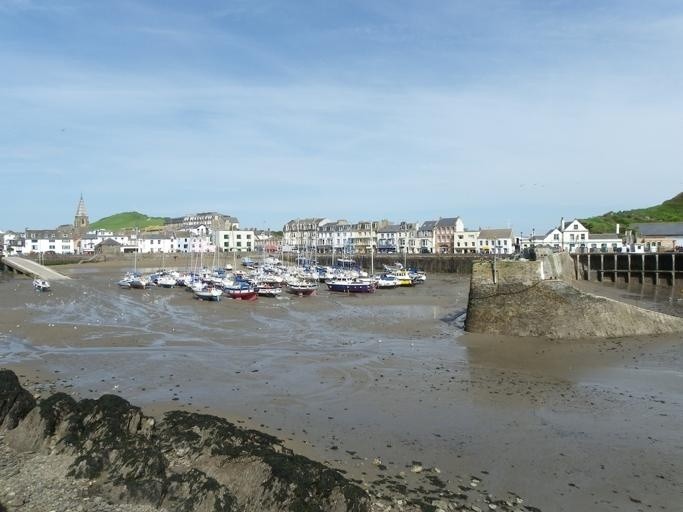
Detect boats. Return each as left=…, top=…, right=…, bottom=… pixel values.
left=32, top=279, right=51, bottom=292
